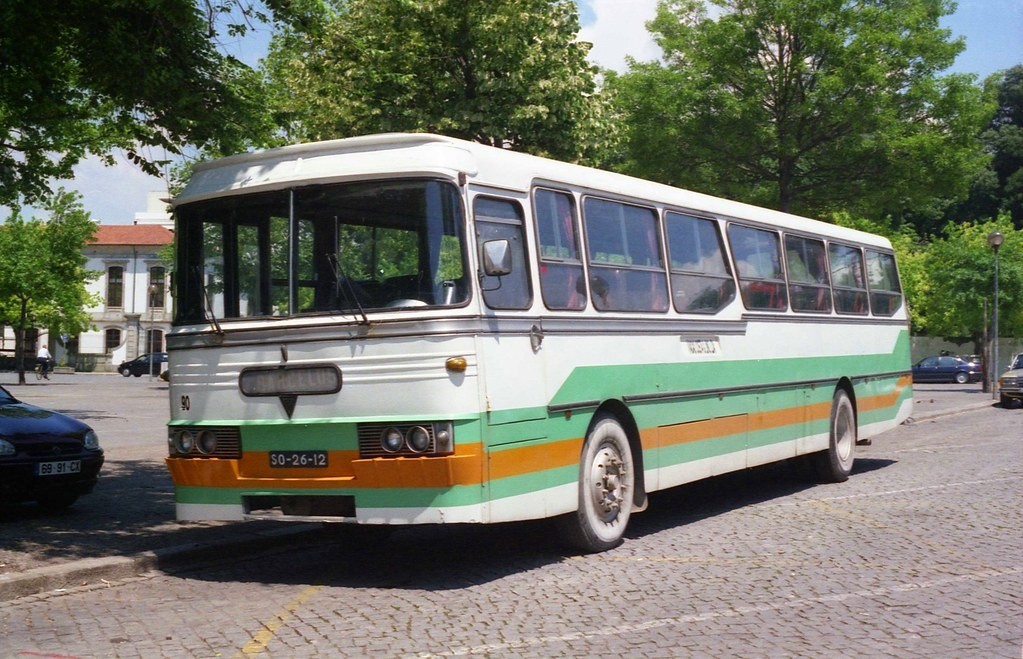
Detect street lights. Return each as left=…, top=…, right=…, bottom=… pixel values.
left=986, top=230, right=1004, bottom=402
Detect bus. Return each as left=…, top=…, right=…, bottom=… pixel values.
left=160, top=128, right=917, bottom=553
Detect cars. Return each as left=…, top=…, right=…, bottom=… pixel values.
left=0, top=385, right=105, bottom=522
left=999, top=350, right=1023, bottom=409
left=912, top=355, right=982, bottom=384
left=117, top=351, right=169, bottom=378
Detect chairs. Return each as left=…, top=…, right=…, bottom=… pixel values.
left=543, top=269, right=899, bottom=313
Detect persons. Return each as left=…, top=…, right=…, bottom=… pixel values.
left=38, top=345, right=51, bottom=380
